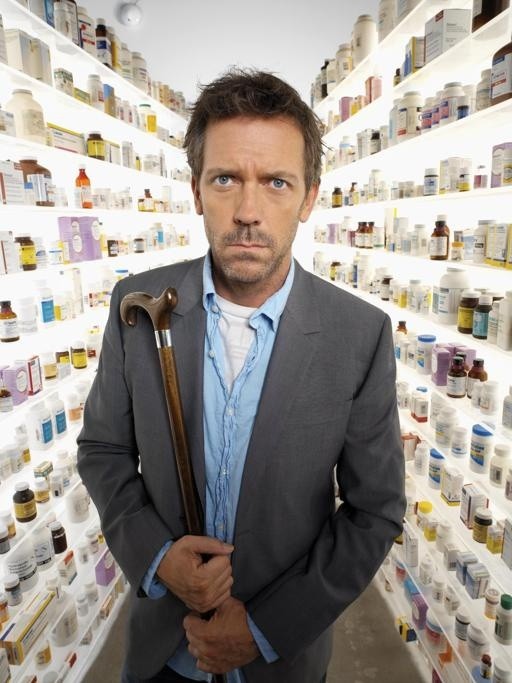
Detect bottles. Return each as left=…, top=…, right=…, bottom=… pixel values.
left=0, top=0, right=195, bottom=682
left=313, top=0, right=512, bottom=682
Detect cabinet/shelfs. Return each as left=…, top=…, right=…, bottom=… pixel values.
left=0, top=0, right=197, bottom=681
left=296, top=0, right=511, bottom=683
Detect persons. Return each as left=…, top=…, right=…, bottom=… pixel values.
left=76, top=64, right=408, bottom=683
